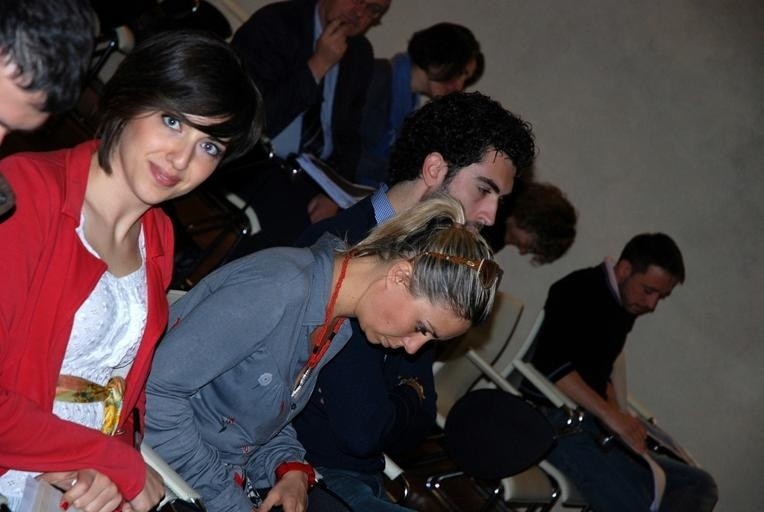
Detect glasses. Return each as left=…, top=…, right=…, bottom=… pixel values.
left=408, top=253, right=506, bottom=289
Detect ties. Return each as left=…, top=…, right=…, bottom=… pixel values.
left=300, top=78, right=324, bottom=157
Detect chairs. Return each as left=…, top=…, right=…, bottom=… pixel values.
left=433, top=290, right=702, bottom=511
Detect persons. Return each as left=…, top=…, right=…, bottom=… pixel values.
left=0, top=1, right=719, bottom=512
